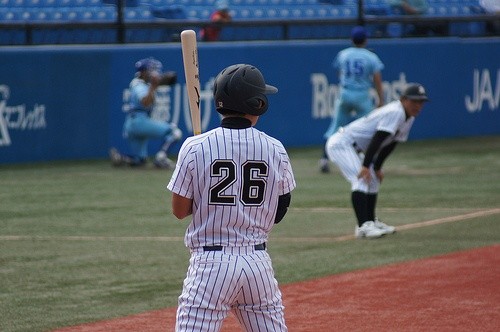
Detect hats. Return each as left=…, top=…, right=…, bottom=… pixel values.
left=402, top=82, right=428, bottom=100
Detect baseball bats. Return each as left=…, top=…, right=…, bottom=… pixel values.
left=180, top=29, right=202, bottom=137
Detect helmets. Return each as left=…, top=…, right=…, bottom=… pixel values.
left=214, top=64, right=277, bottom=115
left=135, top=58, right=162, bottom=79
left=351, top=26, right=370, bottom=43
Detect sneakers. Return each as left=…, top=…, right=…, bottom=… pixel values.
left=354, top=218, right=395, bottom=238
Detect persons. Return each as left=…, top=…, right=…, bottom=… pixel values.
left=166, top=64, right=296, bottom=332
left=479, top=0, right=500, bottom=36
left=325, top=84, right=428, bottom=237
left=110, top=57, right=182, bottom=168
left=320, top=26, right=384, bottom=173
left=385, top=0, right=427, bottom=38
left=197, top=6, right=232, bottom=41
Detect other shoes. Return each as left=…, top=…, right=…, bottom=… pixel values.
left=319, top=158, right=329, bottom=171
left=155, top=157, right=176, bottom=168
left=110, top=147, right=122, bottom=164
